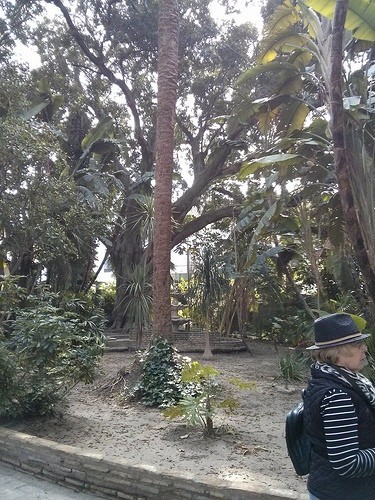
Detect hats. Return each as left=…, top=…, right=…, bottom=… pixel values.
left=305, top=313, right=370, bottom=350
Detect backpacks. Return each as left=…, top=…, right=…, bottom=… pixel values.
left=284, top=388, right=314, bottom=476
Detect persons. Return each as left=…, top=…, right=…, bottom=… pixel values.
left=301, top=314, right=375, bottom=500
